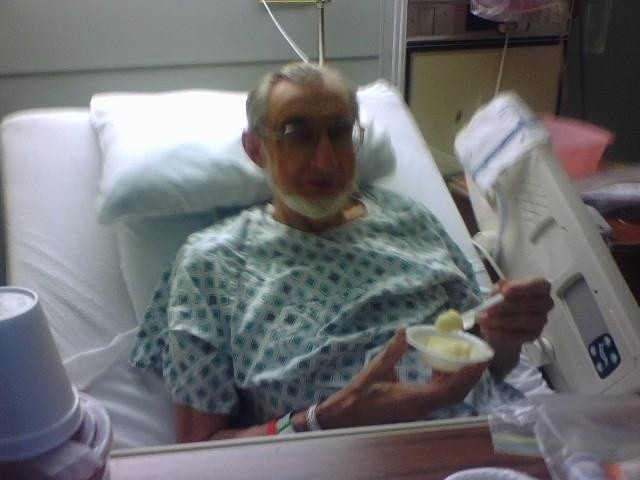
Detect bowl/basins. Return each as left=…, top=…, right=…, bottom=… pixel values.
left=539, top=118, right=612, bottom=177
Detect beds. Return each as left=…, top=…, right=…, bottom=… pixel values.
left=0, top=76, right=640, bottom=453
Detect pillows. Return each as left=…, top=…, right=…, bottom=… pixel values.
left=87, top=79, right=397, bottom=226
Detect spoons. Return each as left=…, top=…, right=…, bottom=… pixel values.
left=461, top=294, right=505, bottom=329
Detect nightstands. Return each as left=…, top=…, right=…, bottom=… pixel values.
left=446, top=151, right=640, bottom=256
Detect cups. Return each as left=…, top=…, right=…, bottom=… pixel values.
left=1, top=287, right=82, bottom=462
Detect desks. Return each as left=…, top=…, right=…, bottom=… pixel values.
left=103, top=414, right=552, bottom=480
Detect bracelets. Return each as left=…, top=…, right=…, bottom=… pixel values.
left=267, top=416, right=278, bottom=435
left=306, top=403, right=325, bottom=432
left=276, top=411, right=298, bottom=436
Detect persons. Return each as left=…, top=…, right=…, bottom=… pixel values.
left=130, top=59, right=555, bottom=445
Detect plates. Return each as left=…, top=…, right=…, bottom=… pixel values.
left=405, top=324, right=494, bottom=374
